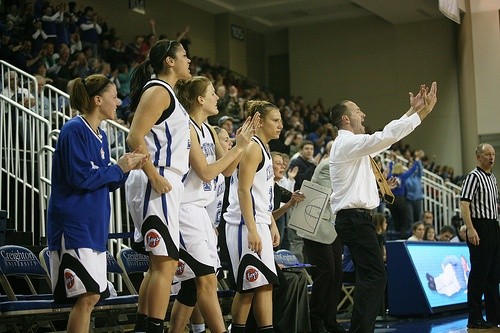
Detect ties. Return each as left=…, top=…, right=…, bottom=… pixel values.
left=368, top=154, right=395, bottom=205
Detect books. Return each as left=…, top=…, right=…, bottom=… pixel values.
left=288, top=181, right=331, bottom=237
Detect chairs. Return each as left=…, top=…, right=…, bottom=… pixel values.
left=0, top=246, right=355, bottom=333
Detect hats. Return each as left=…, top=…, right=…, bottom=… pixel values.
left=219, top=115, right=234, bottom=123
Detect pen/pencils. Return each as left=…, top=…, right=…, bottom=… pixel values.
left=297, top=192, right=302, bottom=195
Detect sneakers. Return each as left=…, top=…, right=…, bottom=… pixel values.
left=465, top=318, right=500, bottom=329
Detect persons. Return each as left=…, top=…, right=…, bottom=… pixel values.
left=48, top=74, right=150, bottom=333
left=460, top=143, right=500, bottom=330
left=328, top=81, right=437, bottom=333
left=223, top=101, right=310, bottom=333
left=124, top=39, right=191, bottom=333
left=0, top=0, right=468, bottom=264
left=297, top=158, right=348, bottom=333
left=173, top=76, right=260, bottom=333
